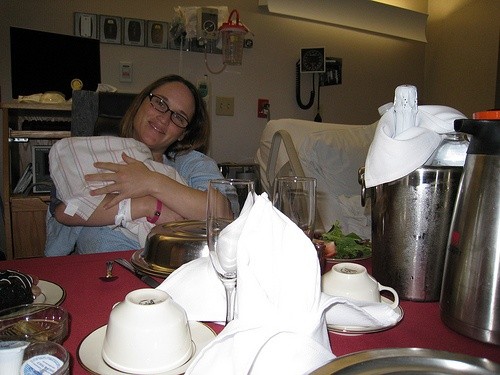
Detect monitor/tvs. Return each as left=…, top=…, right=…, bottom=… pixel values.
left=31, top=145, right=53, bottom=193
left=9, top=26, right=101, bottom=99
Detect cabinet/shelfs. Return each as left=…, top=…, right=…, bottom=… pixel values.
left=0, top=100, right=72, bottom=260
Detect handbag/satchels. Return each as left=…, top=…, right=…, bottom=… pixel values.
left=265, top=130, right=326, bottom=240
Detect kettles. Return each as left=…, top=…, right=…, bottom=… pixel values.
left=438, top=109, right=500, bottom=345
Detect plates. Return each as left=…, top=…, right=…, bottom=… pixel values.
left=77, top=321, right=217, bottom=375
left=327, top=295, right=404, bottom=336
left=0, top=279, right=65, bottom=322
left=325, top=246, right=373, bottom=261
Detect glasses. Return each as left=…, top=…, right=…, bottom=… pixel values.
left=147, top=91, right=191, bottom=130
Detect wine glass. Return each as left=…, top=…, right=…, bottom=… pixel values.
left=207, top=179, right=254, bottom=326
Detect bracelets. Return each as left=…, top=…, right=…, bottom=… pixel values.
left=147, top=200, right=162, bottom=224
left=118, top=198, right=132, bottom=221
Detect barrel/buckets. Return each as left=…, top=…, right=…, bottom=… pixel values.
left=358, top=165, right=464, bottom=303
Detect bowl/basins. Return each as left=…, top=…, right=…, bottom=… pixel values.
left=130, top=219, right=226, bottom=288
left=0, top=304, right=69, bottom=346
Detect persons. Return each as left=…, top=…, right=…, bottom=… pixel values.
left=50, top=74, right=239, bottom=257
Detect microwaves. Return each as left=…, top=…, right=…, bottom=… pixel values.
left=32, top=145, right=52, bottom=193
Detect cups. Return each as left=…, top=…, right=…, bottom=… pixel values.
left=0, top=341, right=24, bottom=375
left=322, top=262, right=399, bottom=309
left=101, top=289, right=196, bottom=375
left=273, top=176, right=317, bottom=241
left=19, top=342, right=70, bottom=375
left=219, top=10, right=248, bottom=66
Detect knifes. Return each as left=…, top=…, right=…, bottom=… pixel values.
left=114, top=257, right=160, bottom=289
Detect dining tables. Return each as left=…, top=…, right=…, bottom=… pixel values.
left=0, top=246, right=500, bottom=374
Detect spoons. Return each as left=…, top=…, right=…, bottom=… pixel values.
left=98, top=260, right=118, bottom=282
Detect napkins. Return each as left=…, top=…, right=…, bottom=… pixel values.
left=365, top=85, right=468, bottom=189
left=158, top=193, right=384, bottom=374
